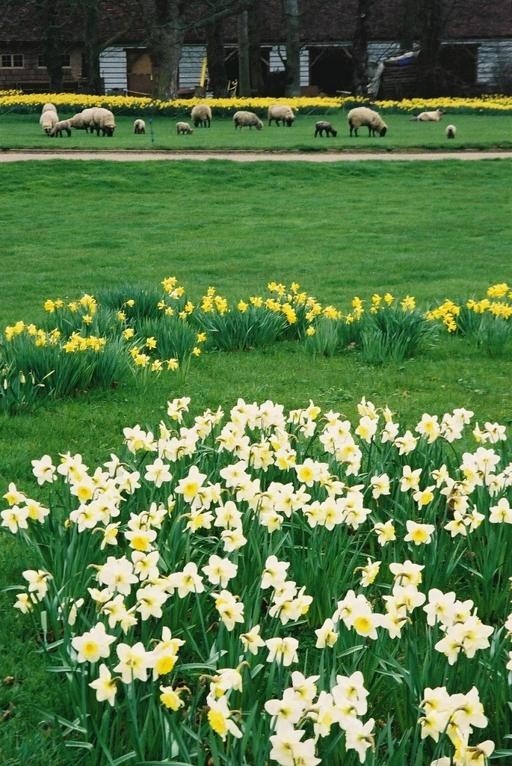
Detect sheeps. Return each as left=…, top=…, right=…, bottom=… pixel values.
left=49, top=120, right=71, bottom=138
left=314, top=121, right=337, bottom=138
left=133, top=118, right=145, bottom=135
left=190, top=105, right=212, bottom=128
left=67, top=106, right=117, bottom=137
left=232, top=110, right=264, bottom=130
left=445, top=124, right=456, bottom=138
left=347, top=106, right=388, bottom=138
left=267, top=105, right=296, bottom=127
left=176, top=122, right=193, bottom=135
left=416, top=108, right=444, bottom=123
left=39, top=102, right=59, bottom=136
